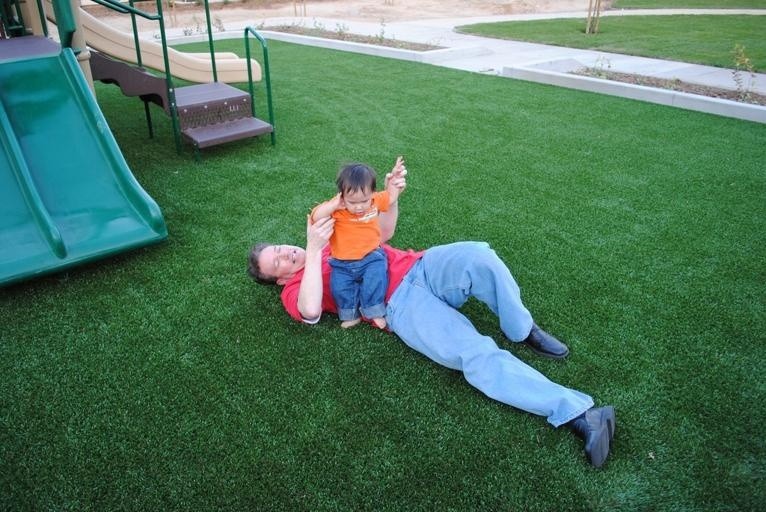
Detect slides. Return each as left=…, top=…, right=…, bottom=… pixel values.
left=39, top=1, right=263, bottom=83
left=0, top=34, right=169, bottom=288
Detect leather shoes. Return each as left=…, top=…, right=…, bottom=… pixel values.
left=568, top=406, right=614, bottom=468
left=523, top=323, right=569, bottom=358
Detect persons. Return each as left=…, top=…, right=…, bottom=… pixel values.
left=308, top=155, right=412, bottom=334
left=247, top=160, right=618, bottom=468
